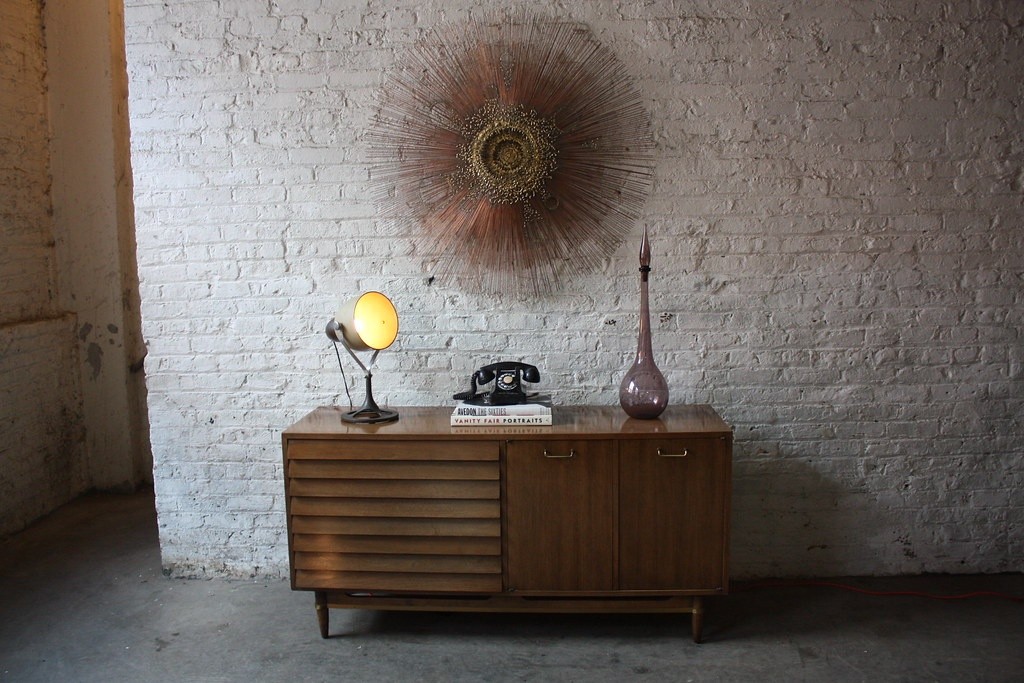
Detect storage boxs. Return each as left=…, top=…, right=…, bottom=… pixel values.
left=280, top=405, right=732, bottom=645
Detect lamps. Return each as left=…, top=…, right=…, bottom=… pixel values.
left=323, top=291, right=400, bottom=424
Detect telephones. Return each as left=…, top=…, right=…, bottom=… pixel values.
left=452, top=361, right=541, bottom=407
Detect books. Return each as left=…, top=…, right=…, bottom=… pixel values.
left=451, top=391, right=553, bottom=426
